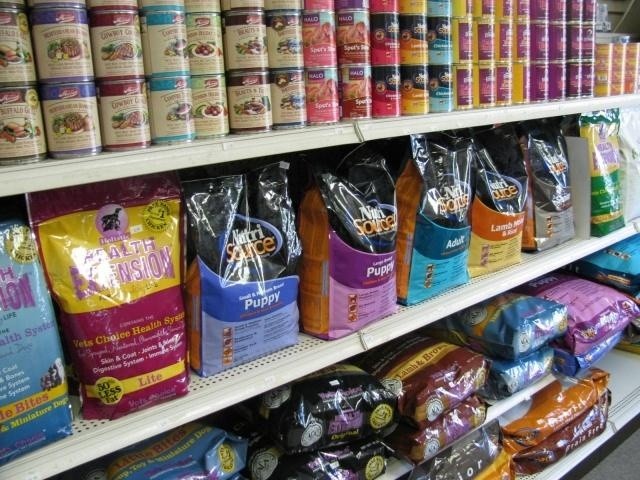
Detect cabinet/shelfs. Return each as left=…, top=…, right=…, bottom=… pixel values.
left=0, top=93, right=640, bottom=480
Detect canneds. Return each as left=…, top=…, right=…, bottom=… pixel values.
left=0, top=1, right=640, bottom=168
